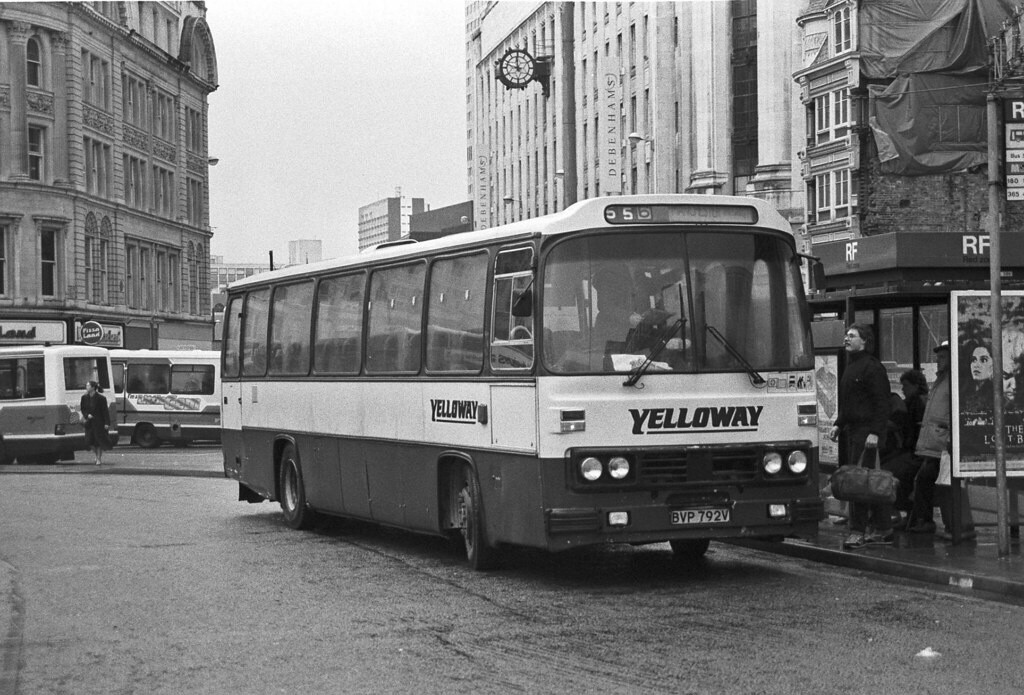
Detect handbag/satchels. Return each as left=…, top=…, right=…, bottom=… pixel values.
left=80, top=416, right=91, bottom=427
left=830, top=446, right=900, bottom=504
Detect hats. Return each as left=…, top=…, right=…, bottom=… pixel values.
left=934, top=345, right=948, bottom=352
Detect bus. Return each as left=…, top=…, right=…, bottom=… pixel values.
left=106, top=348, right=221, bottom=449
left=1, top=344, right=120, bottom=464
left=217, top=192, right=828, bottom=573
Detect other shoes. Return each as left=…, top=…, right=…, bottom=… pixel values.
left=95, top=460, right=101, bottom=466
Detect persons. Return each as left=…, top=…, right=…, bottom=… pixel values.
left=79, top=380, right=111, bottom=466
left=826, top=322, right=895, bottom=551
left=887, top=370, right=940, bottom=533
left=961, top=328, right=1023, bottom=414
left=915, top=339, right=978, bottom=544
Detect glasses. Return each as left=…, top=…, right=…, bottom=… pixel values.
left=844, top=334, right=861, bottom=340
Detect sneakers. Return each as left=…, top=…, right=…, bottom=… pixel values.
left=912, top=521, right=976, bottom=541
left=844, top=530, right=896, bottom=550
